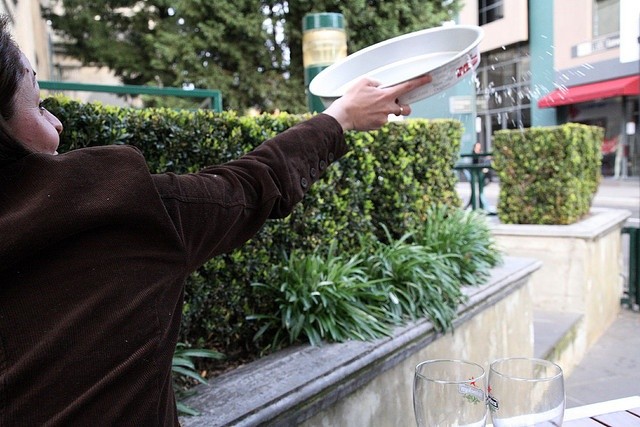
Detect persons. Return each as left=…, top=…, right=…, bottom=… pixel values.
left=474, top=141, right=489, bottom=182
left=1, top=13, right=432, bottom=427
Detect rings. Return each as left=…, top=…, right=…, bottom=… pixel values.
left=396, top=105, right=403, bottom=116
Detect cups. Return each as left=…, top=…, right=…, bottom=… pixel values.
left=411, top=359, right=486, bottom=426
left=488, top=357, right=566, bottom=427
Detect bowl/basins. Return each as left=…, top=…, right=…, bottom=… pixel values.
left=307, top=24, right=483, bottom=109
